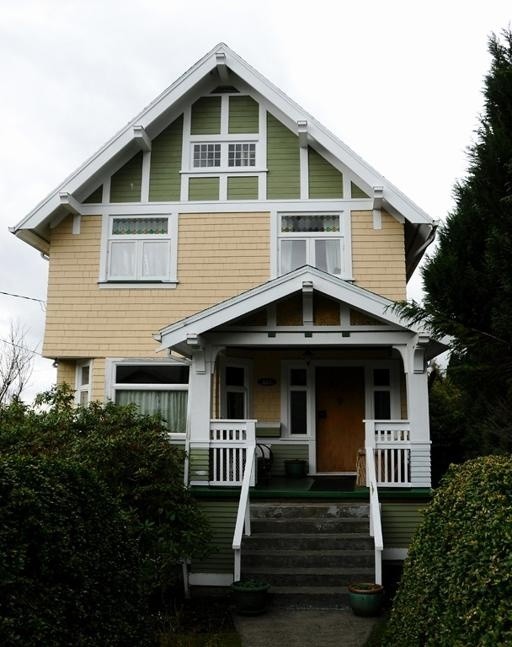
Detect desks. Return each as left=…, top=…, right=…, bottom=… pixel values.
left=355, top=447, right=388, bottom=487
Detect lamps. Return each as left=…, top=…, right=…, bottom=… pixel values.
left=303, top=351, right=313, bottom=368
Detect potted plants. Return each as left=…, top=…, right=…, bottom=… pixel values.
left=230, top=578, right=273, bottom=616
left=347, top=580, right=384, bottom=618
